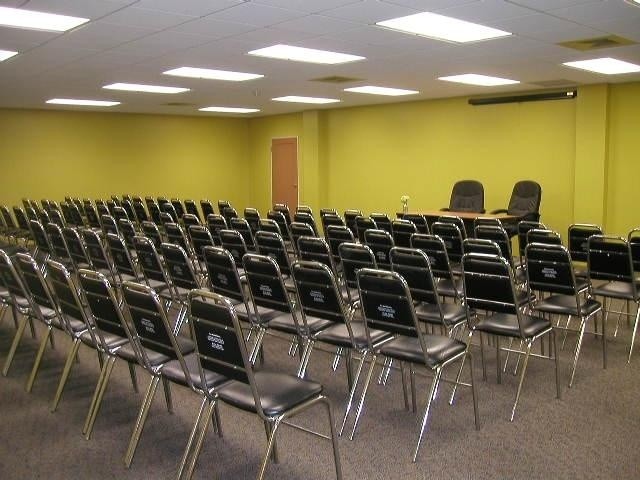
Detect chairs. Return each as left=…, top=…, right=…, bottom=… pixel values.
left=0, top=182, right=639, bottom=479
